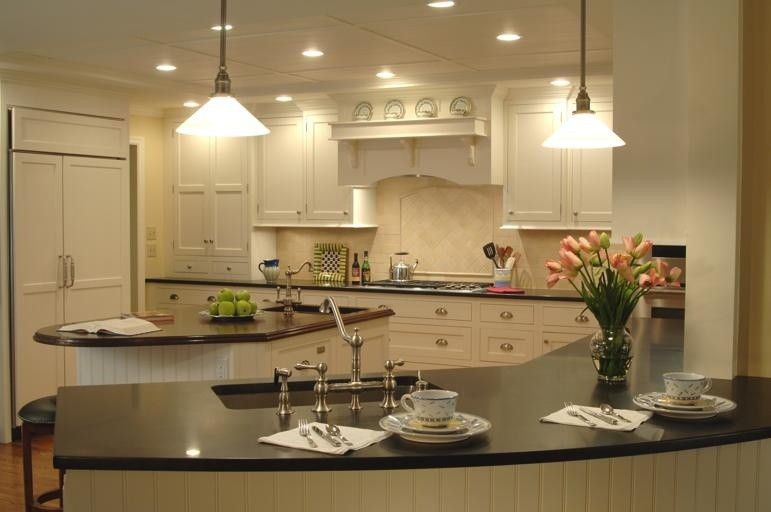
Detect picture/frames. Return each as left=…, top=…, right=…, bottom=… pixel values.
left=312, top=242, right=347, bottom=283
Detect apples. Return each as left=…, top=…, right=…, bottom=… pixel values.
left=209, top=288, right=257, bottom=316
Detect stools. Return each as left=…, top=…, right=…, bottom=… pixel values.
left=16, top=394, right=65, bottom=510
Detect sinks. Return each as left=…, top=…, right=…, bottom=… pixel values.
left=210, top=375, right=447, bottom=411
left=261, top=304, right=369, bottom=314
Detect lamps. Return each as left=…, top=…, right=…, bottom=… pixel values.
left=175, top=0, right=269, bottom=138
left=541, top=1, right=625, bottom=149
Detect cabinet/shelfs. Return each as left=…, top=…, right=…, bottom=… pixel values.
left=167, top=118, right=277, bottom=281
left=354, top=295, right=473, bottom=364
left=271, top=336, right=336, bottom=371
left=540, top=305, right=595, bottom=358
left=570, top=85, right=613, bottom=228
left=157, top=288, right=226, bottom=313
left=253, top=112, right=379, bottom=228
left=244, top=291, right=348, bottom=313
left=502, top=87, right=569, bottom=225
left=6, top=108, right=130, bottom=427
left=479, top=300, right=535, bottom=367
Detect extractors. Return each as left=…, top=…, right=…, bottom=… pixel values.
left=330, top=121, right=502, bottom=188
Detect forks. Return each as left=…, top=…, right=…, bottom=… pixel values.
left=298, top=417, right=319, bottom=449
left=564, top=399, right=597, bottom=428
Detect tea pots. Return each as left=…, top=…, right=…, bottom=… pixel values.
left=258, top=258, right=281, bottom=282
left=389, top=251, right=420, bottom=282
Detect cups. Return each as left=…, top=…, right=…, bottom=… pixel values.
left=494, top=268, right=513, bottom=288
left=662, top=372, right=714, bottom=402
left=400, top=389, right=460, bottom=427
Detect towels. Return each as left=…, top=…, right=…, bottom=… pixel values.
left=540, top=404, right=654, bottom=435
left=258, top=419, right=393, bottom=454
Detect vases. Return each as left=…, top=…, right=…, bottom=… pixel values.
left=588, top=328, right=634, bottom=383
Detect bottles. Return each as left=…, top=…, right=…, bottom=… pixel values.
left=362, top=250, right=371, bottom=284
left=352, top=253, right=360, bottom=284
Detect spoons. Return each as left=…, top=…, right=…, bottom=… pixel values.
left=325, top=423, right=354, bottom=446
left=602, top=402, right=631, bottom=423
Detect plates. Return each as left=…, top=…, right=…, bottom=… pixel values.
left=199, top=309, right=265, bottom=320
left=402, top=419, right=463, bottom=434
left=650, top=392, right=717, bottom=410
left=633, top=391, right=737, bottom=419
left=381, top=411, right=492, bottom=443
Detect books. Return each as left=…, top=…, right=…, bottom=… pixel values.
left=120, top=312, right=176, bottom=324
left=58, top=317, right=162, bottom=335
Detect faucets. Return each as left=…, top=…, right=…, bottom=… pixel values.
left=284, top=260, right=313, bottom=316
left=319, top=295, right=366, bottom=409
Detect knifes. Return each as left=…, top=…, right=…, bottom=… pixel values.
left=581, top=406, right=617, bottom=425
left=313, top=426, right=342, bottom=447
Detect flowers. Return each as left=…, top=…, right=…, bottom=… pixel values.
left=544, top=230, right=683, bottom=376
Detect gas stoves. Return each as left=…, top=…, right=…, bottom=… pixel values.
left=365, top=277, right=482, bottom=291
